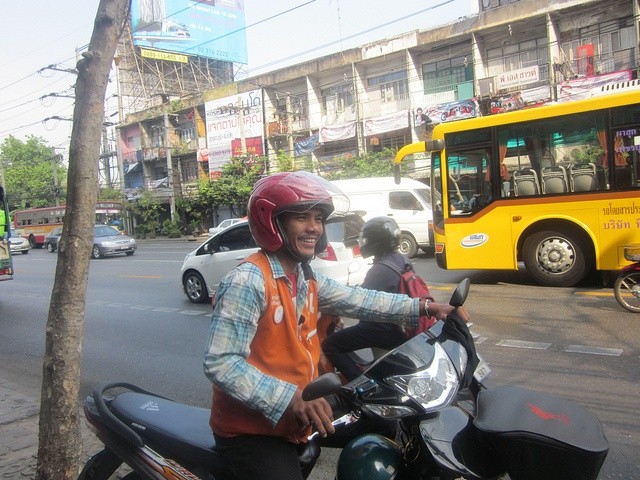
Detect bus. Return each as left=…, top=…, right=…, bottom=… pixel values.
left=14, top=202, right=127, bottom=248
left=393, top=91, right=640, bottom=286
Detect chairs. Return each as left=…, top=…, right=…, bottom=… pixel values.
left=540, top=166, right=569, bottom=193
left=569, top=162, right=599, bottom=193
left=512, top=168, right=541, bottom=195
left=607, top=165, right=633, bottom=189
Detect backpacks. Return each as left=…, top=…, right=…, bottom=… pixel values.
left=373, top=254, right=437, bottom=338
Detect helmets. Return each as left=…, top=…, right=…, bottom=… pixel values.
left=358, top=217, right=401, bottom=258
left=246, top=172, right=335, bottom=253
left=337, top=432, right=403, bottom=480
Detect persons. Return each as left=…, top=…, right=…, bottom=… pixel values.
left=203, top=170, right=471, bottom=479
left=416, top=107, right=432, bottom=125
left=320, top=216, right=416, bottom=383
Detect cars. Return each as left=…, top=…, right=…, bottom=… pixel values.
left=4, top=230, right=32, bottom=254
left=208, top=218, right=243, bottom=238
left=43, top=227, right=64, bottom=252
left=91, top=225, right=137, bottom=259
left=181, top=209, right=374, bottom=304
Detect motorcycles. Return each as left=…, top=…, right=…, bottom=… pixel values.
left=79, top=279, right=611, bottom=480
left=615, top=248, right=640, bottom=312
left=317, top=261, right=491, bottom=397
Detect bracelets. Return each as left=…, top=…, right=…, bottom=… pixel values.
left=424, top=299, right=431, bottom=319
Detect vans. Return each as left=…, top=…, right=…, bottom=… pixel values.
left=329, top=177, right=455, bottom=258
